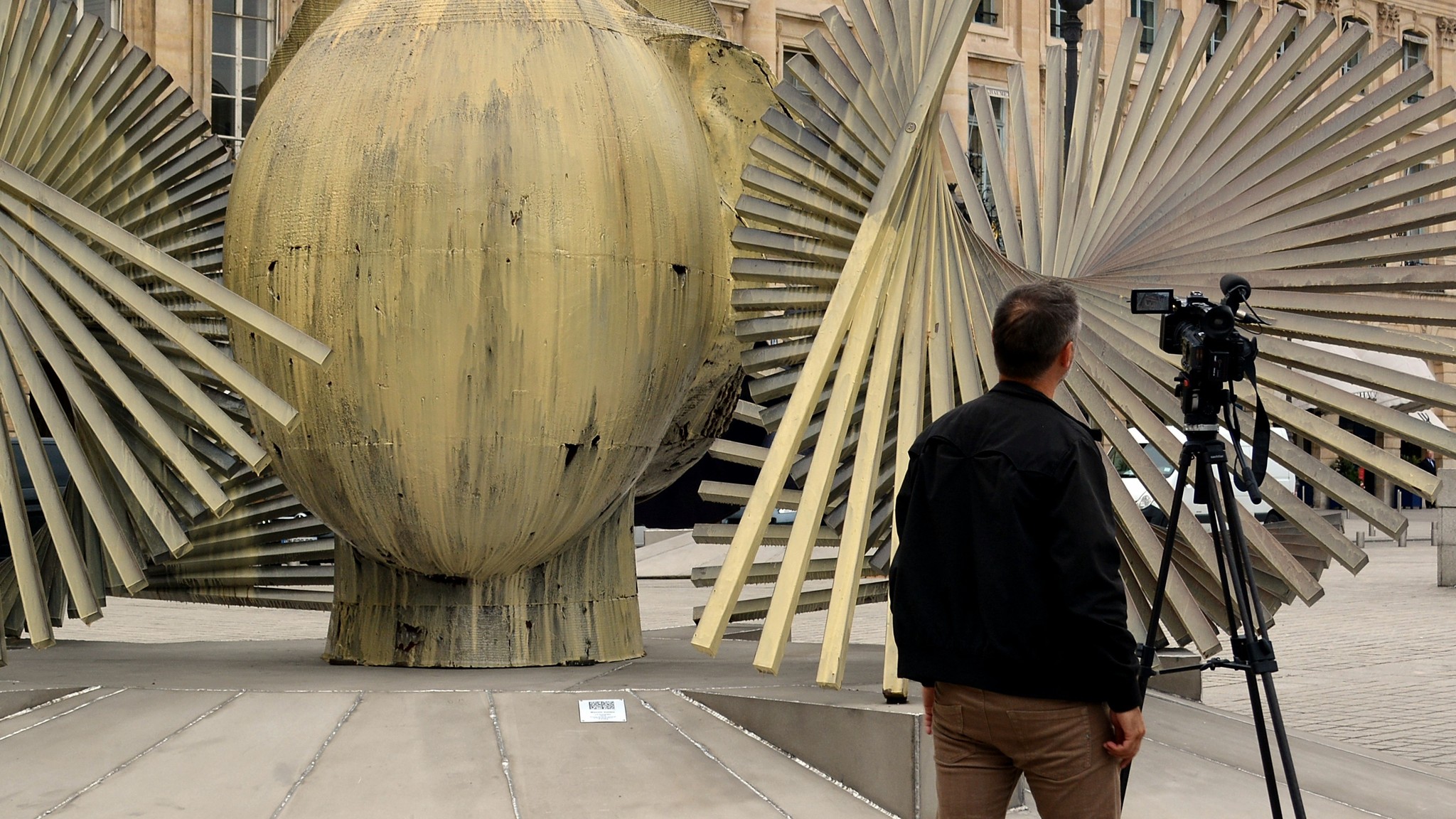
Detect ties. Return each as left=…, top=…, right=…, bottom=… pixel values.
left=1430, top=459, right=1431, bottom=463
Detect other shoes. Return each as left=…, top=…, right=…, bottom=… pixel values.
left=1431, top=506, right=1438, bottom=509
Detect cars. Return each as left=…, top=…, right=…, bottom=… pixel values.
left=0, top=438, right=70, bottom=559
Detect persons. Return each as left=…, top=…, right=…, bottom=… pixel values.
left=1418, top=449, right=1436, bottom=509
left=887, top=278, right=1147, bottom=819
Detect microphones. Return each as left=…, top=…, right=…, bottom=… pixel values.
left=1220, top=274, right=1251, bottom=302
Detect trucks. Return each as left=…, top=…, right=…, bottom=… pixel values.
left=1106, top=425, right=1296, bottom=528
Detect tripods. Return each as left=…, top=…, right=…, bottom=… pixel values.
left=1120, top=373, right=1307, bottom=819
